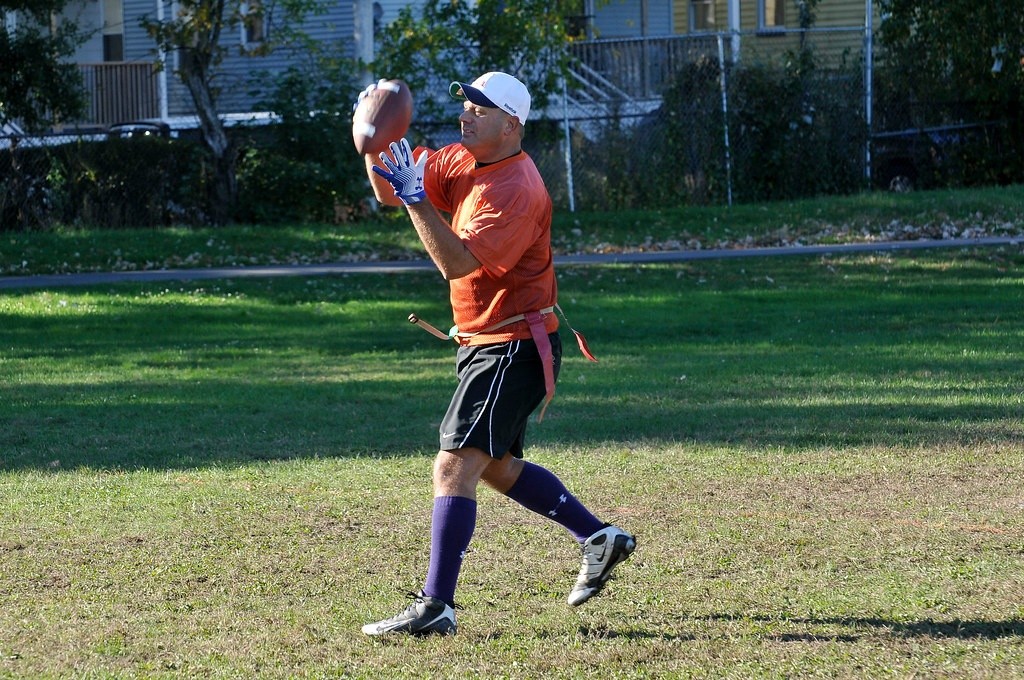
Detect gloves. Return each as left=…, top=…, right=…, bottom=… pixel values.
left=371, top=137, right=428, bottom=205
left=353, top=78, right=387, bottom=111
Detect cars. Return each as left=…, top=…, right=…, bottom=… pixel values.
left=848, top=135, right=948, bottom=197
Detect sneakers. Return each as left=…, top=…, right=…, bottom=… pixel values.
left=568, top=522, right=637, bottom=607
left=361, top=590, right=457, bottom=638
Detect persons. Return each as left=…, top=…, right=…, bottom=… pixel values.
left=351, top=71, right=636, bottom=645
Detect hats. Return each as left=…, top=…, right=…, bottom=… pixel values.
left=449, top=71, right=531, bottom=126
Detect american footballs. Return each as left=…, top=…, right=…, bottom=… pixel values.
left=351, top=78, right=413, bottom=157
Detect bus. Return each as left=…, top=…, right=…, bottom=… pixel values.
left=104, top=111, right=280, bottom=143
